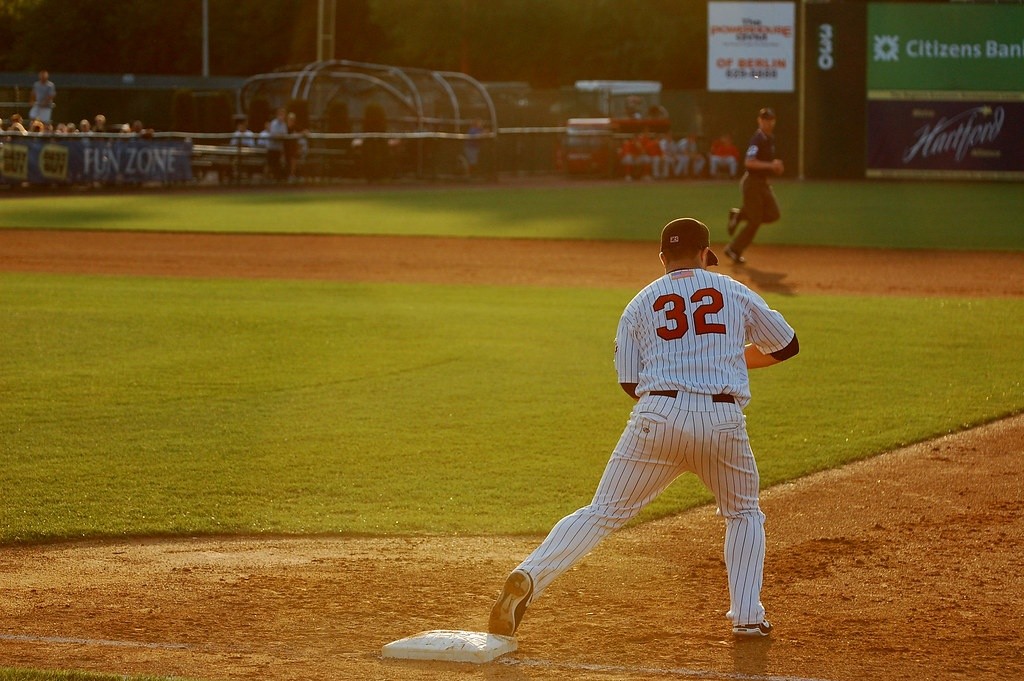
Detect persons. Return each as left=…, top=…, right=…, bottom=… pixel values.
left=463, top=117, right=489, bottom=179
left=0, top=70, right=155, bottom=140
left=488, top=217, right=800, bottom=638
left=710, top=132, right=741, bottom=179
left=723, top=107, right=785, bottom=265
left=621, top=127, right=707, bottom=183
left=230, top=119, right=255, bottom=146
left=258, top=108, right=310, bottom=180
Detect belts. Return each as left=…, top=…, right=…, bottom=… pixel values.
left=648, top=390, right=735, bottom=404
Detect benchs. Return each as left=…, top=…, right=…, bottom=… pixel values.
left=1, top=130, right=367, bottom=196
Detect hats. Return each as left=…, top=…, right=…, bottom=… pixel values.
left=661, top=218, right=718, bottom=266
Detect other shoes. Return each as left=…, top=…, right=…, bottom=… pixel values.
left=724, top=245, right=747, bottom=265
left=727, top=208, right=740, bottom=236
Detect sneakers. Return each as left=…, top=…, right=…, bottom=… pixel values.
left=733, top=619, right=774, bottom=636
left=489, top=568, right=533, bottom=636
